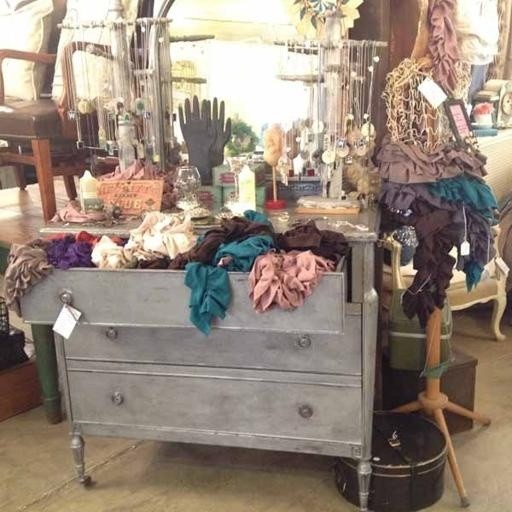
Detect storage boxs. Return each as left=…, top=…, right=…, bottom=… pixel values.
left=382, top=346, right=478, bottom=436
left=387, top=287, right=452, bottom=371
left=333, top=409, right=449, bottom=512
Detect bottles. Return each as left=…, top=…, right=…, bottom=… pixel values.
left=119, top=136, right=135, bottom=172
left=324, top=17, right=342, bottom=42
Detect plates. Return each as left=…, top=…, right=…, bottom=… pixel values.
left=470, top=123, right=492, bottom=129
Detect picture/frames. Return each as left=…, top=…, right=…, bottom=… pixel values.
left=443, top=99, right=476, bottom=145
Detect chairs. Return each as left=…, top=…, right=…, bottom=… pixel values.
left=379, top=220, right=511, bottom=353
left=0, top=0, right=174, bottom=224
left=0, top=0, right=81, bottom=202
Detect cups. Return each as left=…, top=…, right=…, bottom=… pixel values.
left=474, top=114, right=492, bottom=123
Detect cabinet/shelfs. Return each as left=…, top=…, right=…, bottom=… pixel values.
left=17, top=190, right=382, bottom=512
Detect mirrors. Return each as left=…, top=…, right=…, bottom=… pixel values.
left=155, top=0, right=349, bottom=182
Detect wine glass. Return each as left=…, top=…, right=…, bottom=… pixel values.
left=175, top=165, right=202, bottom=206
